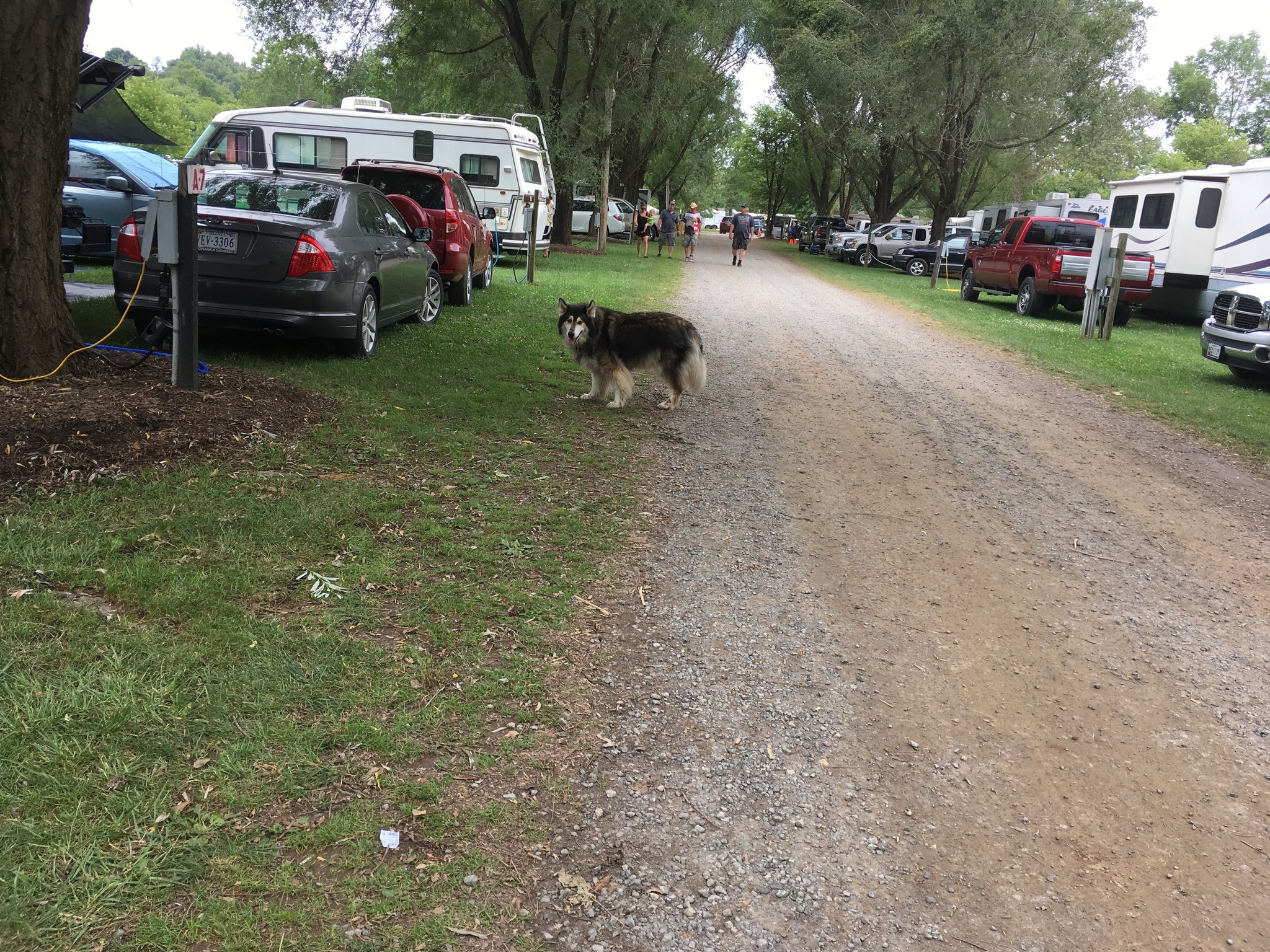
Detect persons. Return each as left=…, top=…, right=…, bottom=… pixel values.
left=640, top=209, right=656, bottom=234
left=681, top=202, right=702, bottom=261
left=657, top=201, right=680, bottom=259
left=729, top=203, right=755, bottom=267
left=634, top=202, right=650, bottom=258
left=543, top=189, right=555, bottom=258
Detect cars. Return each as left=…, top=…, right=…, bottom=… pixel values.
left=570, top=197, right=626, bottom=240
left=753, top=219, right=765, bottom=235
left=58, top=138, right=179, bottom=256
left=110, top=167, right=443, bottom=358
left=719, top=216, right=735, bottom=234
left=609, top=196, right=662, bottom=242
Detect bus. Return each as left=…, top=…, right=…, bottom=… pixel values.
left=1199, top=281, right=1270, bottom=379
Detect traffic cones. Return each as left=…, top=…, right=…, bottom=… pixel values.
left=789, top=239, right=798, bottom=244
left=756, top=227, right=761, bottom=238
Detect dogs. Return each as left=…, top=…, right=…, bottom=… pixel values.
left=551, top=297, right=706, bottom=410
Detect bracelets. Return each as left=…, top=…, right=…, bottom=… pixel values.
left=751, top=237, right=753, bottom=238
left=699, top=232, right=701, bottom=233
left=678, top=231, right=680, bottom=232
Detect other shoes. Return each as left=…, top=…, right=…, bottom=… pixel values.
left=732, top=258, right=737, bottom=265
left=689, top=254, right=693, bottom=262
left=657, top=253, right=662, bottom=257
left=737, top=263, right=742, bottom=267
left=685, top=256, right=689, bottom=261
left=668, top=256, right=673, bottom=259
left=640, top=231, right=646, bottom=234
left=644, top=255, right=648, bottom=258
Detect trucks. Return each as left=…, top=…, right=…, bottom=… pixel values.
left=180, top=97, right=557, bottom=257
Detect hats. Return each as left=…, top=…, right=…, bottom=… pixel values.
left=690, top=202, right=697, bottom=209
left=741, top=203, right=748, bottom=207
left=670, top=201, right=675, bottom=205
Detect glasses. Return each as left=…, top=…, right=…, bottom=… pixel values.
left=741, top=206, right=747, bottom=208
left=641, top=208, right=646, bottom=210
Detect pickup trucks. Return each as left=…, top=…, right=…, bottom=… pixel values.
left=960, top=216, right=1155, bottom=328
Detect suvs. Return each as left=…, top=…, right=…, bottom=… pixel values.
left=338, top=157, right=497, bottom=309
left=797, top=215, right=997, bottom=278
